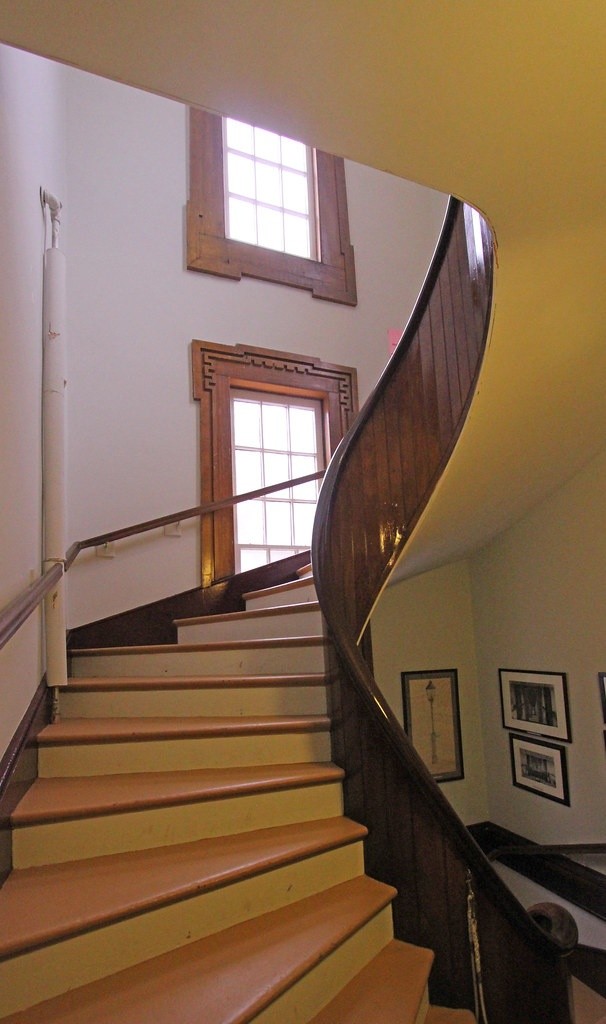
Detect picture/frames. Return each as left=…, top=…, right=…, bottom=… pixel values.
left=498, top=668, right=573, bottom=744
left=401, top=669, right=465, bottom=783
left=598, top=671, right=606, bottom=724
left=508, top=732, right=571, bottom=808
